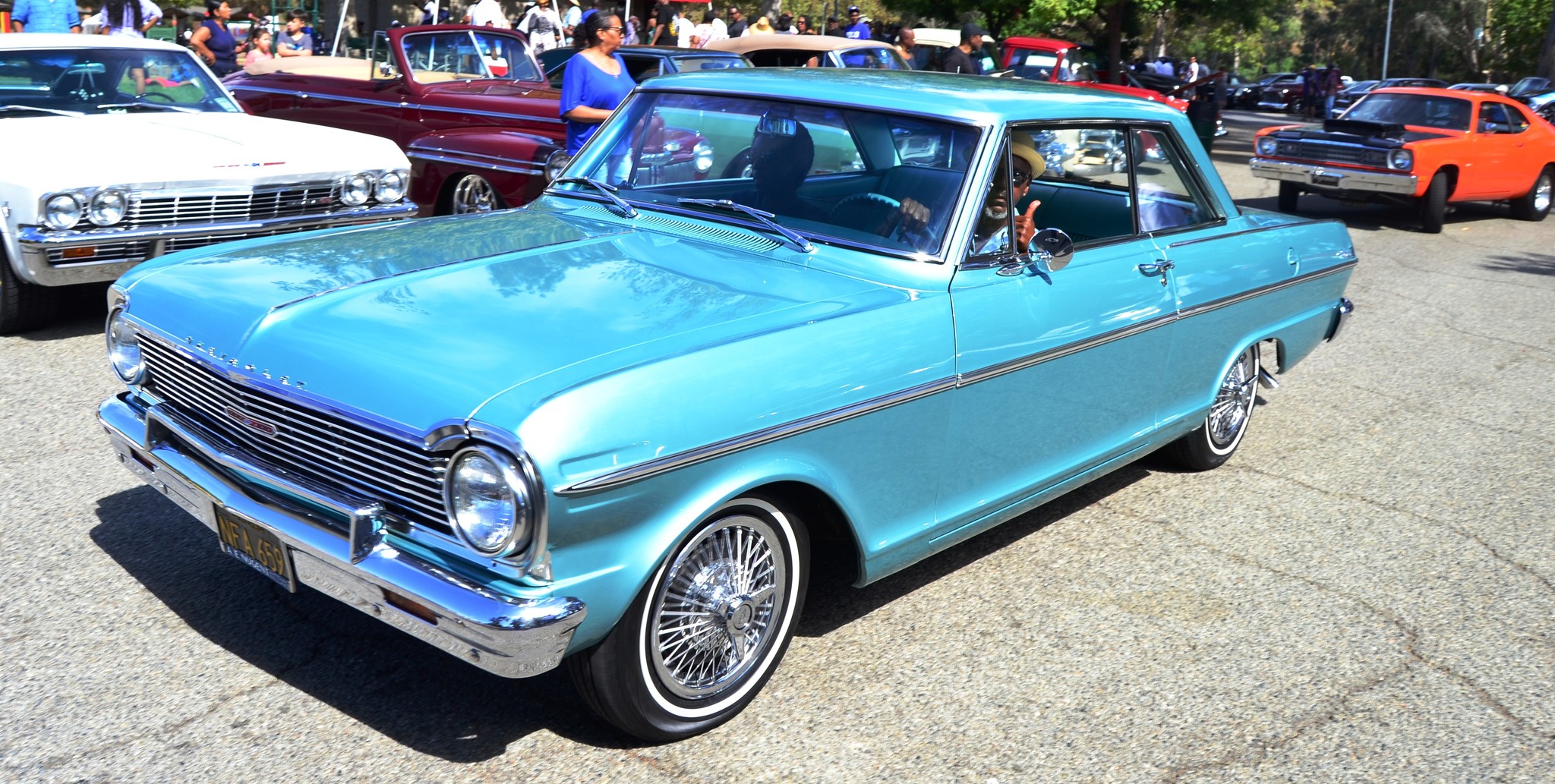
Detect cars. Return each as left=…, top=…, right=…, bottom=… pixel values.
left=96, top=65, right=1361, bottom=747
left=533, top=28, right=1555, bottom=179
left=0, top=31, right=424, bottom=336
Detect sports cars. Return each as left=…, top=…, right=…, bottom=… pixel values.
left=1249, top=86, right=1555, bottom=234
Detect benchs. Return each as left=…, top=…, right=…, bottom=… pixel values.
left=868, top=164, right=1192, bottom=241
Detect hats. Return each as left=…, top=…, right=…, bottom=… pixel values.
left=828, top=14, right=838, bottom=22
left=749, top=16, right=776, bottom=36
left=569, top=0, right=581, bottom=7
left=848, top=5, right=860, bottom=13
left=1307, top=64, right=1316, bottom=69
left=962, top=131, right=1046, bottom=179
left=961, top=23, right=990, bottom=39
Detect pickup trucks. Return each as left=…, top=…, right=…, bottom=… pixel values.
left=219, top=23, right=716, bottom=218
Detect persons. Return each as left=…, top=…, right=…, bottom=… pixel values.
left=243, top=27, right=273, bottom=67
left=942, top=23, right=991, bottom=73
left=1138, top=52, right=1229, bottom=110
left=191, top=1, right=244, bottom=78
left=559, top=11, right=637, bottom=186
left=463, top=0, right=511, bottom=76
left=1491, top=85, right=1510, bottom=123
left=1320, top=64, right=1339, bottom=120
left=647, top=0, right=918, bottom=71
left=276, top=8, right=312, bottom=58
left=516, top=0, right=642, bottom=55
left=872, top=130, right=1041, bottom=255
left=101, top=0, right=164, bottom=98
left=728, top=118, right=832, bottom=224
left=1297, top=63, right=1322, bottom=122
left=412, top=0, right=434, bottom=25
left=11, top=0, right=81, bottom=85
left=128, top=65, right=200, bottom=89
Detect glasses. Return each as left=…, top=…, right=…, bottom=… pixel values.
left=1013, top=171, right=1029, bottom=184
left=798, top=20, right=806, bottom=25
left=601, top=26, right=624, bottom=32
left=745, top=150, right=781, bottom=165
left=729, top=11, right=738, bottom=15
